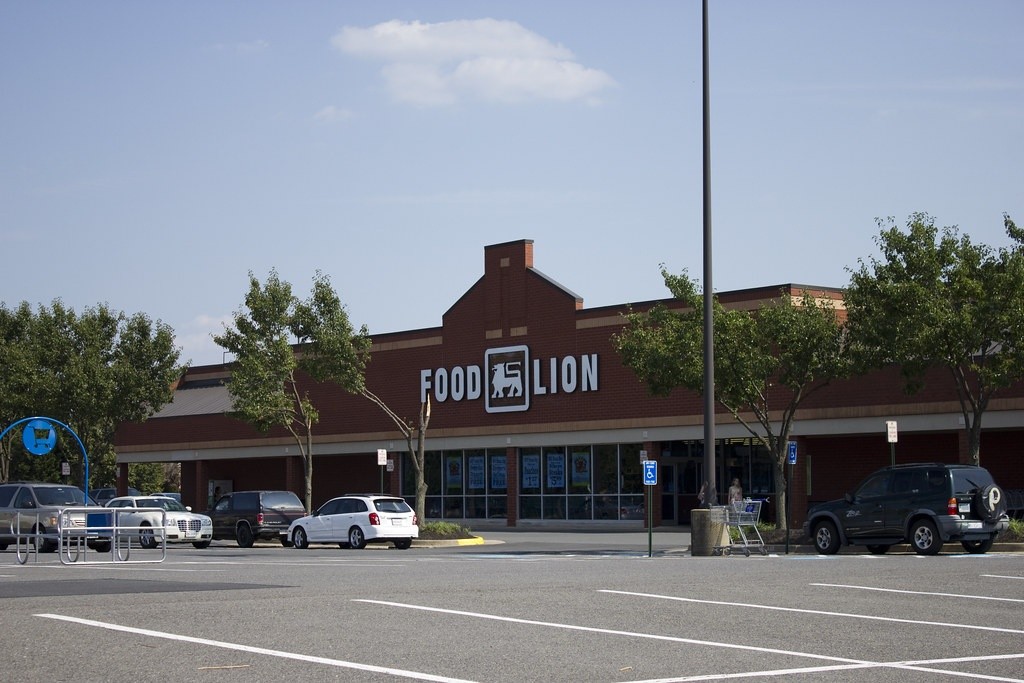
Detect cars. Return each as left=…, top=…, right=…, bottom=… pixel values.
left=149, top=492, right=181, bottom=503
left=87, top=495, right=213, bottom=551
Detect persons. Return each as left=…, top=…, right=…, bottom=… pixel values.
left=728, top=479, right=742, bottom=518
left=214, top=486, right=222, bottom=505
left=697, top=484, right=704, bottom=509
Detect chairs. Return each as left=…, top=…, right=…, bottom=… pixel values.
left=335, top=501, right=344, bottom=513
left=343, top=503, right=352, bottom=513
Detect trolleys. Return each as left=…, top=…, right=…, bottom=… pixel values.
left=707, top=496, right=767, bottom=557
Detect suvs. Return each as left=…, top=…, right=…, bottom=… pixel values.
left=802, top=462, right=1011, bottom=555
left=199, top=490, right=306, bottom=548
left=87, top=487, right=141, bottom=508
left=285, top=493, right=419, bottom=550
left=1, top=480, right=112, bottom=553
